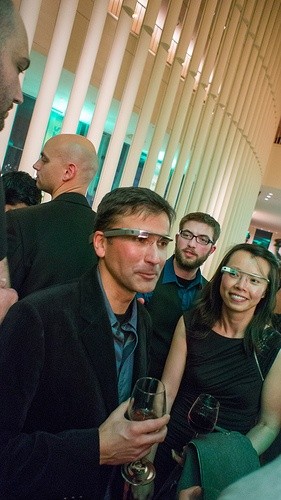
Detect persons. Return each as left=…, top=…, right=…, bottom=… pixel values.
left=0, top=134, right=281, bottom=500
left=0, top=0, right=31, bottom=327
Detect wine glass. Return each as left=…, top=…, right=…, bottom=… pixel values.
left=175, top=393, right=220, bottom=458
left=120, top=377, right=167, bottom=487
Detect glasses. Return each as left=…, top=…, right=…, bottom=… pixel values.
left=179, top=229, right=215, bottom=245
